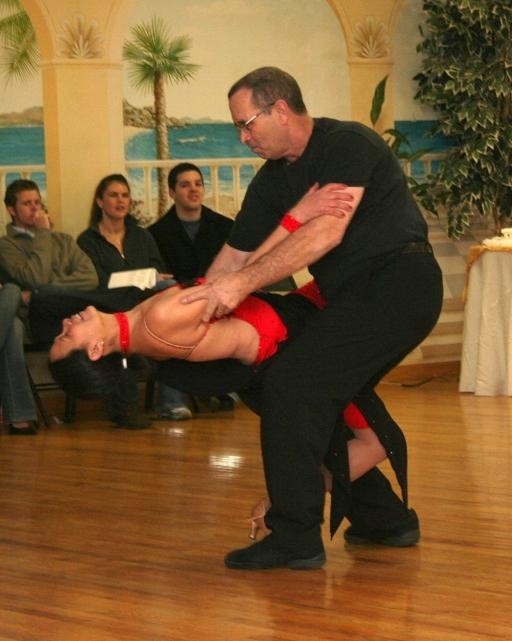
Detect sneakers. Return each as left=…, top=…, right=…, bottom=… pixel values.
left=159, top=406, right=192, bottom=420
left=345, top=508, right=420, bottom=546
left=193, top=391, right=240, bottom=412
left=225, top=526, right=327, bottom=569
left=111, top=412, right=152, bottom=428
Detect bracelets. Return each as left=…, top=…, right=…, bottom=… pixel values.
left=279, top=211, right=303, bottom=235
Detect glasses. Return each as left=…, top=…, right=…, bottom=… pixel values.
left=235, top=104, right=275, bottom=129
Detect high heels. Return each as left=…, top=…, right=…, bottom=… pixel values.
left=8, top=424, right=35, bottom=434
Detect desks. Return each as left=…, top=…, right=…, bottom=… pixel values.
left=455, top=240, right=512, bottom=398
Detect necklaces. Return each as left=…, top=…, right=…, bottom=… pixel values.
left=113, top=311, right=133, bottom=356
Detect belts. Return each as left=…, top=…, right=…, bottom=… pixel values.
left=397, top=241, right=433, bottom=256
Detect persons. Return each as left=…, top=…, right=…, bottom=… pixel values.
left=0, top=276, right=39, bottom=435
left=147, top=162, right=239, bottom=417
left=75, top=173, right=195, bottom=423
left=0, top=177, right=154, bottom=432
left=42, top=180, right=394, bottom=541
left=175, top=66, right=447, bottom=573
